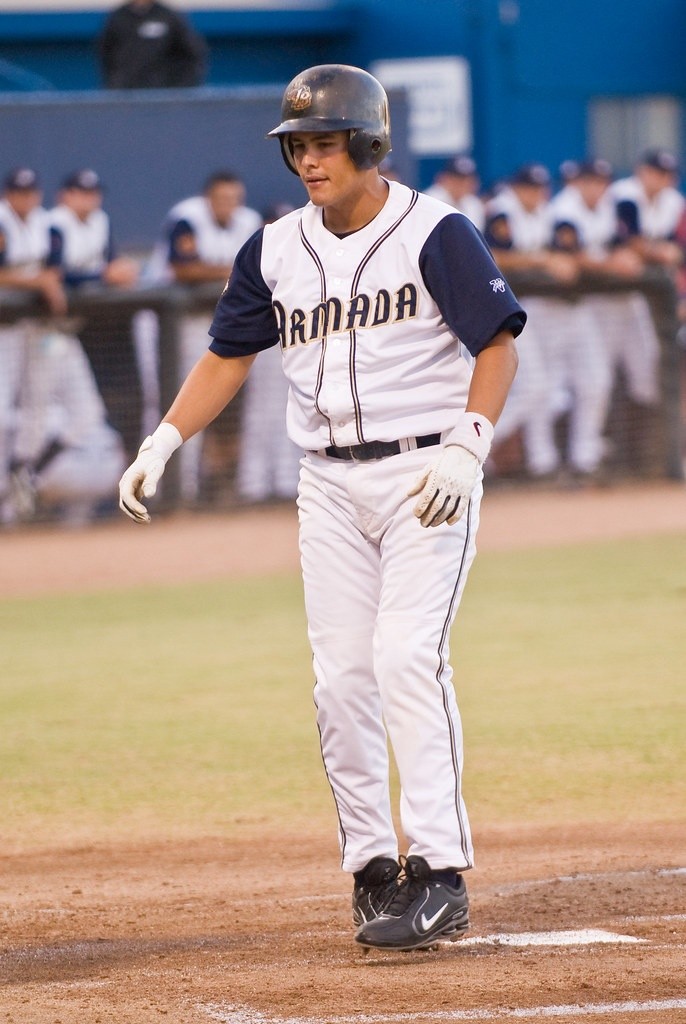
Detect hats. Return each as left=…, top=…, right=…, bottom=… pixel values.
left=644, top=148, right=677, bottom=170
left=580, top=156, right=611, bottom=176
left=5, top=170, right=38, bottom=190
left=515, top=163, right=548, bottom=186
left=443, top=156, right=476, bottom=176
left=64, top=170, right=100, bottom=190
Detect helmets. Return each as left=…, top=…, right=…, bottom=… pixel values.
left=265, top=65, right=393, bottom=176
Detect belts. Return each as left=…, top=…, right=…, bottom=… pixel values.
left=307, top=432, right=440, bottom=460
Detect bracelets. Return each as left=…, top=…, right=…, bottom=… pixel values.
left=442, top=412, right=495, bottom=463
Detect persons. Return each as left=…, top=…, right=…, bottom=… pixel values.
left=98, top=0, right=200, bottom=89
left=0, top=167, right=307, bottom=525
left=123, top=65, right=530, bottom=952
left=378, top=147, right=686, bottom=482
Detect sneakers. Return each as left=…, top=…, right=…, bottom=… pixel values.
left=352, top=856, right=403, bottom=927
left=354, top=856, right=469, bottom=950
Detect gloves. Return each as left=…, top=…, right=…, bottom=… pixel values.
left=118, top=423, right=183, bottom=524
left=408, top=445, right=479, bottom=529
